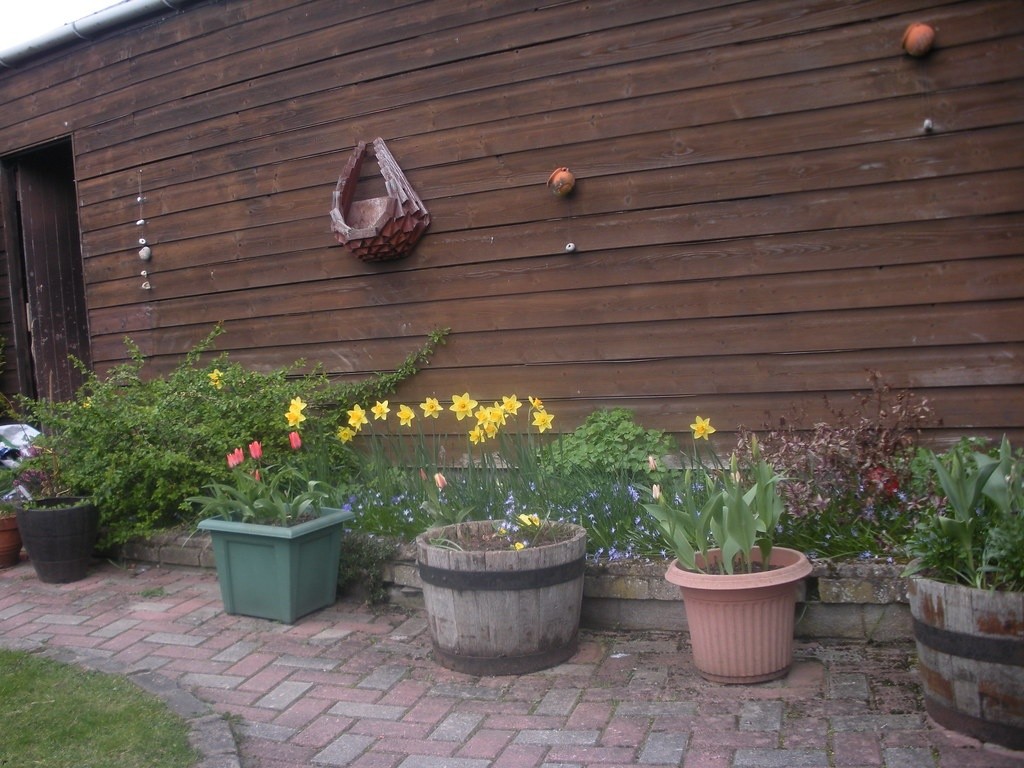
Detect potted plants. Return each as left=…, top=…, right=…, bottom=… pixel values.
left=0, top=467, right=23, bottom=570
left=900, top=432, right=1024, bottom=751
left=0, top=393, right=99, bottom=584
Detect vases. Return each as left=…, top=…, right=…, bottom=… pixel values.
left=195, top=504, right=356, bottom=625
left=416, top=515, right=588, bottom=677
left=664, top=546, right=814, bottom=685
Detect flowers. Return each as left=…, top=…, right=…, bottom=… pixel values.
left=637, top=415, right=803, bottom=575
left=420, top=497, right=582, bottom=550
left=184, top=430, right=341, bottom=526
